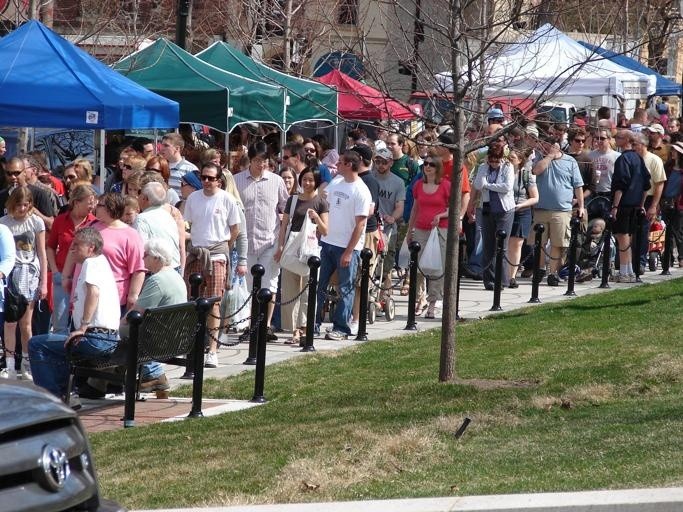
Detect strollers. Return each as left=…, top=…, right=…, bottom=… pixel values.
left=320, top=220, right=398, bottom=325
left=572, top=193, right=614, bottom=282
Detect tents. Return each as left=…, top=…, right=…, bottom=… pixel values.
left=0, top=18, right=179, bottom=195
left=93, top=37, right=422, bottom=179
left=432, top=23, right=657, bottom=126
left=577, top=40, right=682, bottom=97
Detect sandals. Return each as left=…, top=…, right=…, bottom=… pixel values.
left=400, top=281, right=409, bottom=295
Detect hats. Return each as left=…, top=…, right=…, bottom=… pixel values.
left=670, top=142, right=683, bottom=154
left=489, top=109, right=503, bottom=119
left=375, top=149, right=393, bottom=160
left=524, top=126, right=539, bottom=137
left=647, top=124, right=665, bottom=134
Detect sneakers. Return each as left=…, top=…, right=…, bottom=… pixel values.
left=205, top=352, right=217, bottom=368
left=66, top=393, right=82, bottom=409
left=22, top=369, right=32, bottom=381
left=0, top=368, right=17, bottom=381
left=140, top=374, right=169, bottom=393
left=483, top=256, right=683, bottom=291
left=243, top=320, right=358, bottom=344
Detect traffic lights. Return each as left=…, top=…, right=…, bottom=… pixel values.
left=395, top=59, right=412, bottom=76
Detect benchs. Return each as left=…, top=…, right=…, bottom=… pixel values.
left=64, top=295, right=223, bottom=428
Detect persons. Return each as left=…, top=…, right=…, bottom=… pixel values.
left=461, top=96, right=682, bottom=291
left=2, top=122, right=471, bottom=410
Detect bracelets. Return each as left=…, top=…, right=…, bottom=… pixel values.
left=612, top=206, right=618, bottom=209
left=81, top=319, right=90, bottom=325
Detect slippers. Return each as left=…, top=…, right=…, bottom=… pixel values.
left=415, top=303, right=427, bottom=316
left=424, top=309, right=435, bottom=319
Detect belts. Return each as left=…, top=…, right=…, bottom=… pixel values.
left=86, top=327, right=118, bottom=336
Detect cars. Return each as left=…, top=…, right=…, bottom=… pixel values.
left=533, top=99, right=580, bottom=147
left=0, top=371, right=128, bottom=511
left=0, top=118, right=217, bottom=179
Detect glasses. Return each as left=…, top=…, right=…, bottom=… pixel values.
left=597, top=137, right=606, bottom=140
left=5, top=169, right=24, bottom=176
left=305, top=148, right=315, bottom=153
left=181, top=182, right=189, bottom=187
left=424, top=161, right=436, bottom=167
left=283, top=155, right=295, bottom=159
left=376, top=161, right=390, bottom=165
left=489, top=159, right=501, bottom=163
left=64, top=175, right=75, bottom=179
left=575, top=139, right=585, bottom=143
left=653, top=146, right=662, bottom=151
left=201, top=175, right=217, bottom=181
left=123, top=164, right=132, bottom=170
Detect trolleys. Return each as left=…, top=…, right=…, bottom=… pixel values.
left=646, top=206, right=667, bottom=273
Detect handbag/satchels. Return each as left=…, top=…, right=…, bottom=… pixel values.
left=4, top=284, right=28, bottom=322
